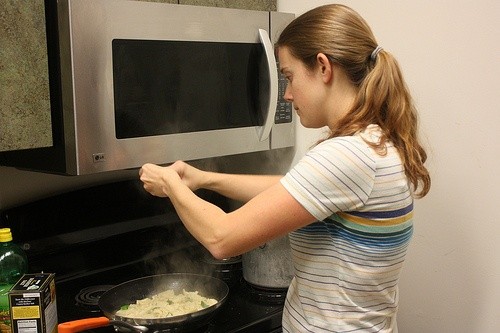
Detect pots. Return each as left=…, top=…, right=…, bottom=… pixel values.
left=57, top=272, right=230, bottom=333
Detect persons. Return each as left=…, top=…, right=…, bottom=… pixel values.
left=139, top=4, right=430, bottom=333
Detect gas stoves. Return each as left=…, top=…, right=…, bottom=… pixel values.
left=53, top=239, right=287, bottom=333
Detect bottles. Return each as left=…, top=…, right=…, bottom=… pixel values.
left=0, top=228, right=30, bottom=333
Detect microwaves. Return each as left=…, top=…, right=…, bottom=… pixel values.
left=44, top=0, right=295, bottom=177
